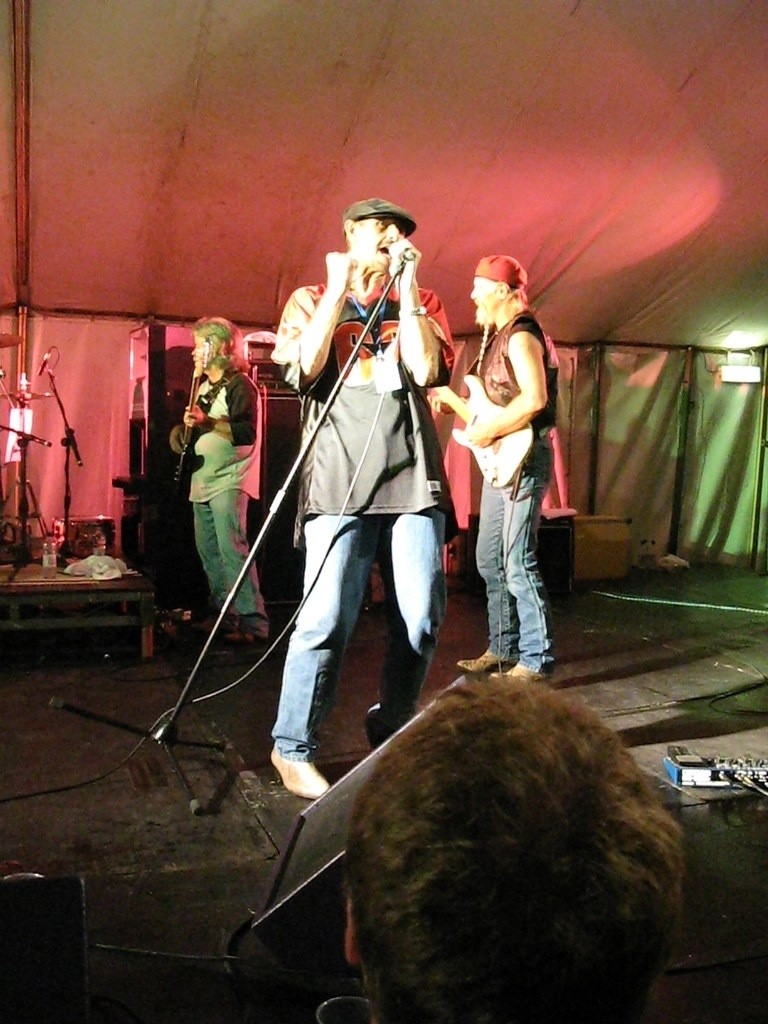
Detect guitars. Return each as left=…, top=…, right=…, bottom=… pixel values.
left=172, top=341, right=211, bottom=482
left=432, top=373, right=535, bottom=490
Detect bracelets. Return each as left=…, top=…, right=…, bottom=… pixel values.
left=399, top=306, right=427, bottom=318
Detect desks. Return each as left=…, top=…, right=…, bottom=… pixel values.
left=0, top=575, right=158, bottom=657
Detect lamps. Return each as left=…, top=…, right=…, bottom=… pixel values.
left=718, top=365, right=761, bottom=383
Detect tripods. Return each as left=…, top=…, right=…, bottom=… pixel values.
left=47, top=256, right=410, bottom=815
left=0, top=399, right=48, bottom=552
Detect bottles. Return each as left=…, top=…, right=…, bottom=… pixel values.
left=42, top=533, right=57, bottom=579
left=92, top=526, right=106, bottom=556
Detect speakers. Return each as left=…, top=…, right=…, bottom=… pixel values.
left=125, top=319, right=312, bottom=614
left=466, top=513, right=574, bottom=594
left=253, top=675, right=479, bottom=977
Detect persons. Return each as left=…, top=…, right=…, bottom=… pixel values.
left=169, top=316, right=266, bottom=643
left=270, top=198, right=458, bottom=800
left=343, top=677, right=685, bottom=1024
left=427, top=254, right=557, bottom=683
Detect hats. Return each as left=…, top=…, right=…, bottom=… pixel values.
left=340, top=197, right=417, bottom=238
left=473, top=255, right=529, bottom=291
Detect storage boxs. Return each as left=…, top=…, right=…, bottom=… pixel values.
left=52, top=516, right=116, bottom=546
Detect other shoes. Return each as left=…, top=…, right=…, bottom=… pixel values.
left=270, top=750, right=330, bottom=798
left=489, top=663, right=549, bottom=683
left=365, top=703, right=390, bottom=752
left=457, top=647, right=509, bottom=673
left=224, top=629, right=257, bottom=642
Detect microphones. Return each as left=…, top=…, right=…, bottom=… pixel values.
left=389, top=243, right=415, bottom=263
left=36, top=351, right=50, bottom=376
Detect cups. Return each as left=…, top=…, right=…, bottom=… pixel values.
left=315, top=996, right=372, bottom=1024
